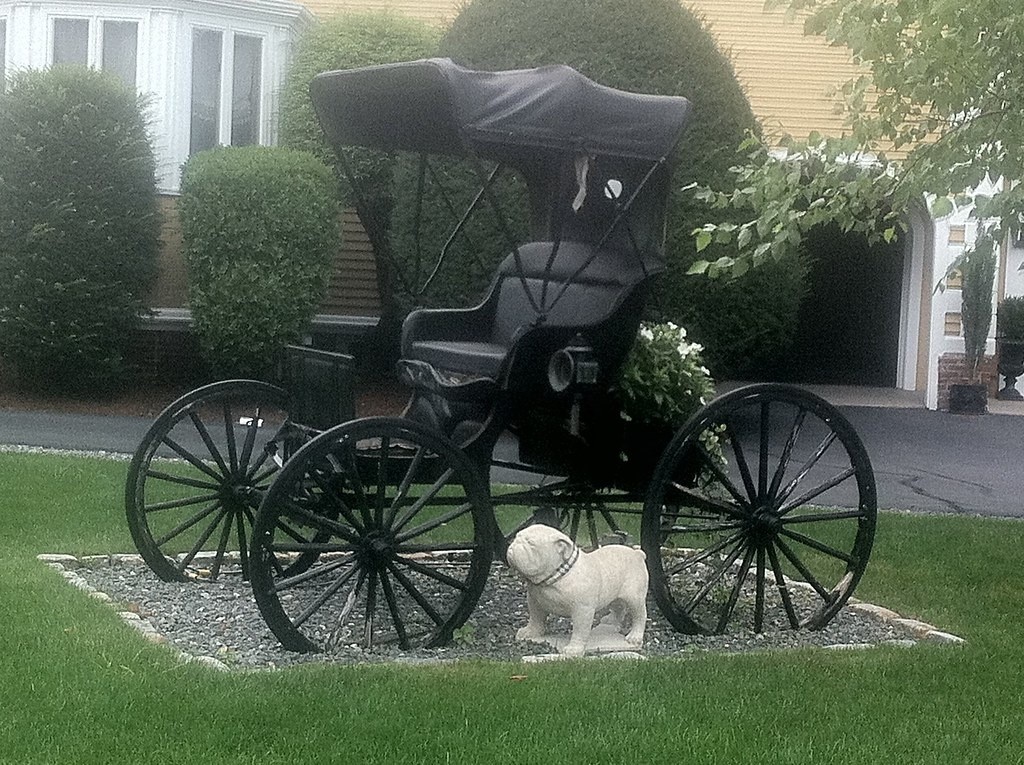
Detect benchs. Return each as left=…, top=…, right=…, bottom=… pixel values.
left=400, top=238, right=667, bottom=383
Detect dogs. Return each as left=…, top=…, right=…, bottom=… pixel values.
left=506, top=523, right=649, bottom=654
